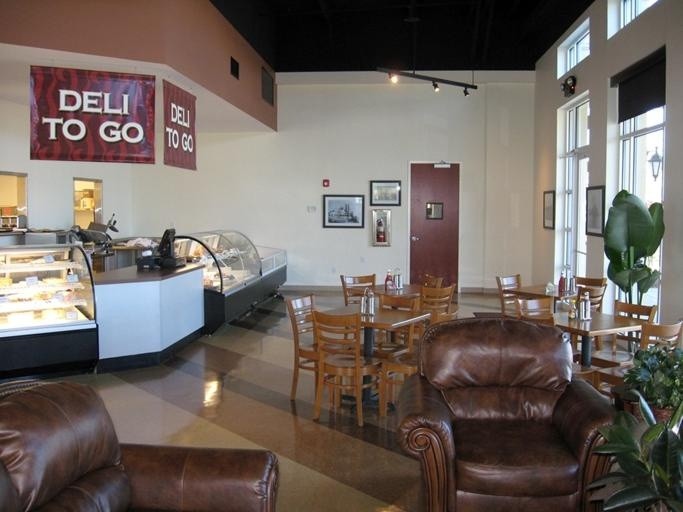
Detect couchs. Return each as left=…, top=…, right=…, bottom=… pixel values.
left=393, top=315, right=618, bottom=512
left=0, top=379, right=280, bottom=512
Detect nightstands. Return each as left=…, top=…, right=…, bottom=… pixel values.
left=0, top=241, right=99, bottom=379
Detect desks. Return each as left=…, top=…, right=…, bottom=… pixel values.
left=531, top=307, right=653, bottom=383
left=347, top=283, right=433, bottom=351
left=326, top=302, right=429, bottom=411
left=504, top=281, right=597, bottom=322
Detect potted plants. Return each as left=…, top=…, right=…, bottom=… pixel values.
left=619, top=342, right=683, bottom=425
left=587, top=398, right=683, bottom=512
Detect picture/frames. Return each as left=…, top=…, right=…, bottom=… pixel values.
left=543, top=192, right=557, bottom=229
left=425, top=202, right=443, bottom=220
left=371, top=208, right=392, bottom=247
left=368, top=180, right=403, bottom=205
left=323, top=193, right=365, bottom=229
left=585, top=187, right=606, bottom=237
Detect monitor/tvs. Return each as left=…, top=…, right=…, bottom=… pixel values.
left=154, top=228, right=186, bottom=260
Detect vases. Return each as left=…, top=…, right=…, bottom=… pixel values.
left=602, top=187, right=665, bottom=354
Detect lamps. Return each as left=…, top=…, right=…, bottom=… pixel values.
left=433, top=81, right=441, bottom=94
left=388, top=75, right=401, bottom=84
left=650, top=146, right=662, bottom=181
left=463, top=87, right=472, bottom=98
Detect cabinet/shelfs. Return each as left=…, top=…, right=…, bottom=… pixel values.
left=253, top=242, right=288, bottom=305
left=90, top=256, right=209, bottom=369
left=174, top=229, right=262, bottom=340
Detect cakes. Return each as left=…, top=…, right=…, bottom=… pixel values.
left=14, top=280, right=44, bottom=301
left=42, top=278, right=67, bottom=290
left=57, top=291, right=76, bottom=306
left=42, top=309, right=73, bottom=324
left=0, top=276, right=12, bottom=295
left=213, top=272, right=238, bottom=286
left=5, top=311, right=33, bottom=329
left=204, top=279, right=213, bottom=288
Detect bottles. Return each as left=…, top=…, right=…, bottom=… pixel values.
left=568, top=300, right=576, bottom=318
left=385, top=269, right=393, bottom=291
left=360, top=288, right=375, bottom=317
left=580, top=292, right=591, bottom=320
left=559, top=273, right=565, bottom=292
left=393, top=268, right=402, bottom=290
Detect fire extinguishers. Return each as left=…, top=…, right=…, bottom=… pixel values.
left=377, top=217, right=385, bottom=242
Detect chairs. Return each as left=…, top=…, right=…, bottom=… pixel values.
left=591, top=300, right=658, bottom=376
left=576, top=284, right=607, bottom=318
left=495, top=274, right=534, bottom=316
left=419, top=275, right=443, bottom=285
left=598, top=320, right=683, bottom=397
left=573, top=274, right=607, bottom=350
left=340, top=274, right=376, bottom=309
left=285, top=293, right=321, bottom=404
left=421, top=285, right=453, bottom=322
left=311, top=309, right=384, bottom=428
left=382, top=295, right=421, bottom=364
left=388, top=312, right=459, bottom=394
